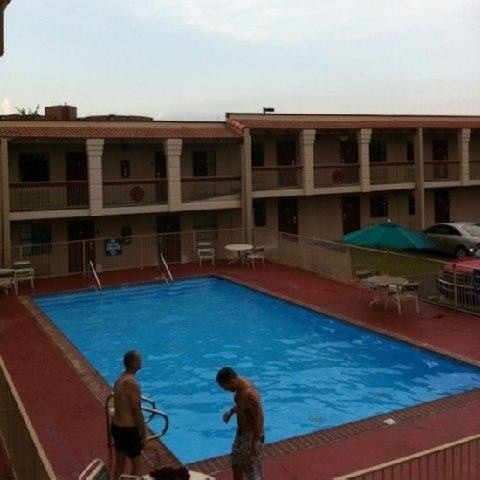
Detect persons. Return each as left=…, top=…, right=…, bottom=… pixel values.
left=111, top=350, right=148, bottom=480
left=216, top=366, right=266, bottom=480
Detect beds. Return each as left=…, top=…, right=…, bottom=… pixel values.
left=341, top=221, right=438, bottom=252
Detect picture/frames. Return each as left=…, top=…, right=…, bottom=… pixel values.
left=224, top=243, right=253, bottom=267
left=142, top=469, right=216, bottom=480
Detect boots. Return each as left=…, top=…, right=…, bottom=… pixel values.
left=437, top=248, right=480, bottom=303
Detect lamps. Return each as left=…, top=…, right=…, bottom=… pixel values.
left=245, top=244, right=265, bottom=271
left=353, top=271, right=419, bottom=315
left=197, top=241, right=214, bottom=268
left=0, top=260, right=34, bottom=296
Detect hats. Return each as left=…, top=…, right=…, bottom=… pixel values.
left=423, top=221, right=480, bottom=258
